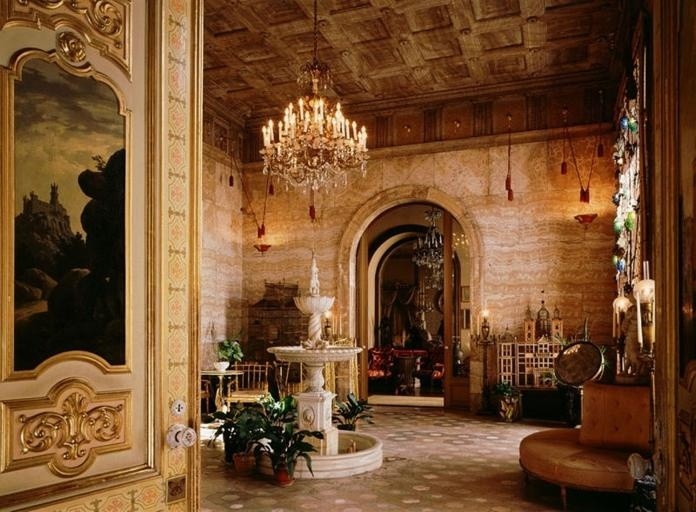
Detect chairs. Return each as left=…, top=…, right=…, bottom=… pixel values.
left=201, top=378, right=211, bottom=414
left=224, top=360, right=269, bottom=412
left=429, top=346, right=445, bottom=394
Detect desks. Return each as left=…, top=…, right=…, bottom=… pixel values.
left=201, top=369, right=244, bottom=414
left=392, top=349, right=427, bottom=393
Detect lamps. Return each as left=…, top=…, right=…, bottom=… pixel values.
left=411, top=206, right=444, bottom=271
left=259, top=0, right=371, bottom=194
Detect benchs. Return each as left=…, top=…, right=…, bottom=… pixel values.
left=519, top=428, right=654, bottom=511
left=369, top=348, right=393, bottom=392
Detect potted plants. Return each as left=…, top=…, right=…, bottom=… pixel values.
left=214, top=340, right=244, bottom=370
left=201, top=392, right=324, bottom=488
left=332, top=391, right=375, bottom=430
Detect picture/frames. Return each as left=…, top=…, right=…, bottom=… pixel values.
left=0, top=28, right=137, bottom=385
left=461, top=285, right=470, bottom=303
